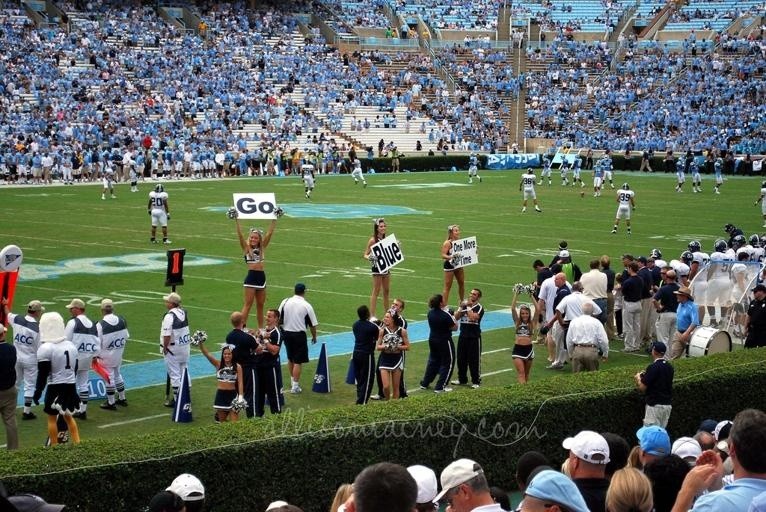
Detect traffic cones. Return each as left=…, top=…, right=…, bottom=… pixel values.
left=266, top=354, right=285, bottom=409
left=345, top=351, right=360, bottom=384
left=171, top=368, right=193, bottom=423
left=311, top=342, right=332, bottom=394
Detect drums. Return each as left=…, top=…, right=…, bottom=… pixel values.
left=685, top=324, right=731, bottom=358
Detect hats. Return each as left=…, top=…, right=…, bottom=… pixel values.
left=100, top=299, right=114, bottom=310
left=163, top=292, right=182, bottom=305
left=750, top=285, right=766, bottom=293
left=65, top=299, right=86, bottom=310
left=405, top=418, right=735, bottom=512
left=558, top=250, right=570, bottom=258
left=7, top=492, right=67, bottom=511
left=27, top=300, right=46, bottom=311
left=295, top=283, right=305, bottom=292
left=632, top=256, right=647, bottom=265
left=672, top=286, right=694, bottom=302
left=651, top=341, right=666, bottom=355
left=164, top=473, right=205, bottom=503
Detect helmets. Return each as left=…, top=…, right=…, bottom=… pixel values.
left=527, top=167, right=533, bottom=174
left=680, top=251, right=693, bottom=261
left=649, top=249, right=662, bottom=259
left=688, top=240, right=701, bottom=252
left=542, top=153, right=609, bottom=164
left=622, top=183, right=629, bottom=190
left=714, top=224, right=766, bottom=253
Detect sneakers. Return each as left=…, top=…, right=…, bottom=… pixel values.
left=627, top=231, right=631, bottom=234
left=370, top=394, right=384, bottom=400
left=545, top=363, right=565, bottom=371
left=100, top=400, right=117, bottom=410
left=0, top=174, right=223, bottom=184
left=72, top=411, right=87, bottom=419
left=291, top=387, right=303, bottom=393
left=116, top=398, right=128, bottom=407
left=421, top=380, right=480, bottom=392
left=22, top=412, right=36, bottom=420
left=675, top=186, right=721, bottom=195
left=164, top=401, right=175, bottom=408
left=611, top=230, right=617, bottom=233
left=536, top=181, right=615, bottom=189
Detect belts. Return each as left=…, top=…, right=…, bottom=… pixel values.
left=575, top=344, right=596, bottom=347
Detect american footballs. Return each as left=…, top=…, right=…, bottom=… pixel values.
left=580, top=191, right=584, bottom=195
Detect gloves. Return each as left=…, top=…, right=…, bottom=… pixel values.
left=32, top=391, right=43, bottom=405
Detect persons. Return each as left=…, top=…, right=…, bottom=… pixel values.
left=0, top=0, right=766, bottom=511
